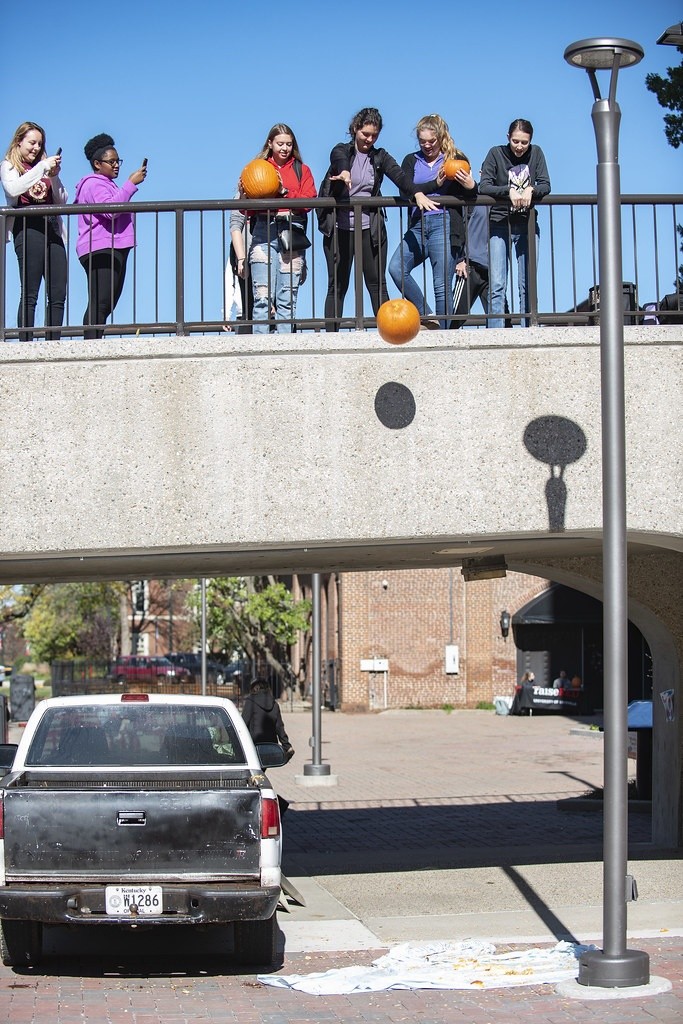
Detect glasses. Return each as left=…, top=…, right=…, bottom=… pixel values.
left=99, top=159, right=122, bottom=167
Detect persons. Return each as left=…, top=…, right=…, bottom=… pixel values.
left=223, top=126, right=311, bottom=337
left=521, top=670, right=540, bottom=687
left=242, top=678, right=295, bottom=774
left=71, top=134, right=147, bottom=342
left=0, top=122, right=68, bottom=341
left=479, top=120, right=551, bottom=328
left=315, top=108, right=441, bottom=333
left=450, top=162, right=514, bottom=328
left=390, top=112, right=481, bottom=328
left=553, top=671, right=572, bottom=688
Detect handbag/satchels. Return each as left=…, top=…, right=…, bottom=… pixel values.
left=276, top=218, right=311, bottom=251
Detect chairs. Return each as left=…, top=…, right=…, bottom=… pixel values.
left=162, top=724, right=212, bottom=763
left=55, top=727, right=109, bottom=764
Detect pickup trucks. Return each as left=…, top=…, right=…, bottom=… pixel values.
left=0, top=694, right=104, bottom=780
left=0, top=692, right=288, bottom=975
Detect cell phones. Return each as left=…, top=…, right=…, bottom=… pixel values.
left=142, top=158, right=148, bottom=169
left=56, top=147, right=62, bottom=156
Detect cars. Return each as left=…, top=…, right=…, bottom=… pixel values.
left=164, top=651, right=226, bottom=688
left=224, top=658, right=284, bottom=698
left=110, top=654, right=191, bottom=687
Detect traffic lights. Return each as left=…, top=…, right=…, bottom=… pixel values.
left=132, top=580, right=151, bottom=612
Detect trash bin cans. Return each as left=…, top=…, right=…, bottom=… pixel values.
left=10, top=675, right=34, bottom=722
left=0, top=695, right=8, bottom=744
left=588, top=282, right=636, bottom=325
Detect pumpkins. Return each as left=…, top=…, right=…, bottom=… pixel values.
left=377, top=297, right=420, bottom=344
left=241, top=159, right=279, bottom=199
left=444, top=159, right=470, bottom=180
left=572, top=675, right=581, bottom=687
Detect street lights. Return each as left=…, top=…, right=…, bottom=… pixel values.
left=559, top=35, right=652, bottom=988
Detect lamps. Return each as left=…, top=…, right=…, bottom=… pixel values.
left=461, top=554, right=507, bottom=583
left=501, top=611, right=510, bottom=636
